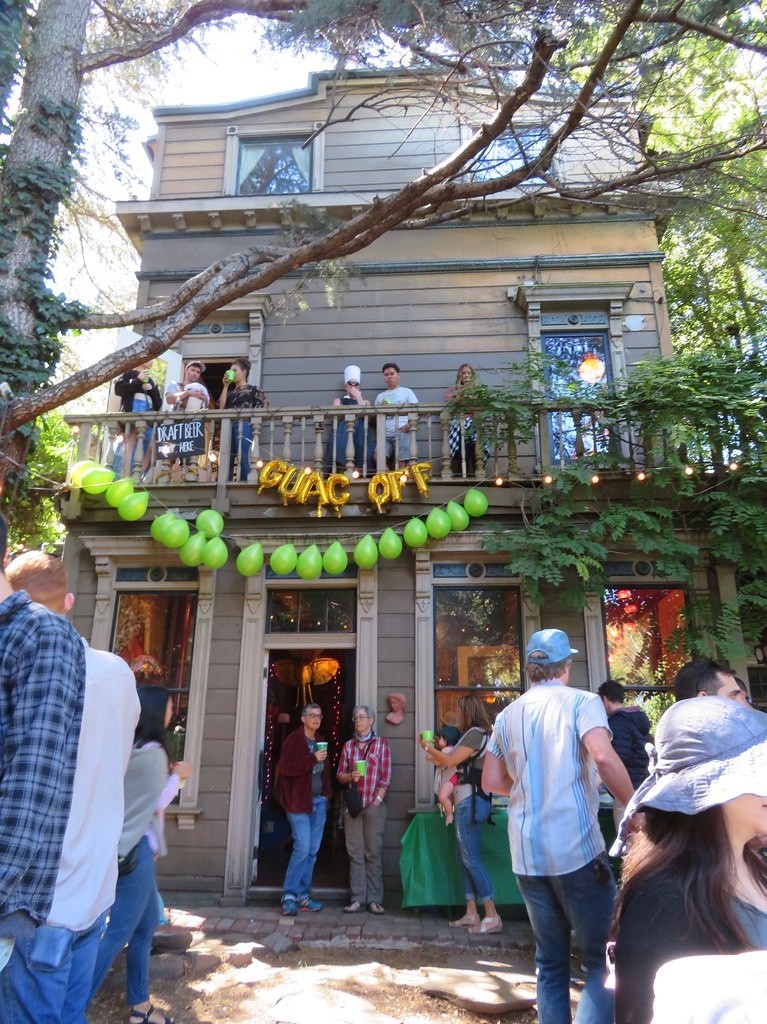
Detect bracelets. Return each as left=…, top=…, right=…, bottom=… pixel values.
left=376, top=795, right=382, bottom=802
left=425, top=744, right=430, bottom=752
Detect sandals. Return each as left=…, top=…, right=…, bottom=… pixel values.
left=468, top=915, right=502, bottom=934
left=449, top=913, right=481, bottom=928
left=129, top=1005, right=174, bottom=1024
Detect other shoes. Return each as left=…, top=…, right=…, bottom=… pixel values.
left=369, top=903, right=384, bottom=913
left=344, top=901, right=366, bottom=912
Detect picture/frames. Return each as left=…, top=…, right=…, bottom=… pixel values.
left=451, top=638, right=520, bottom=695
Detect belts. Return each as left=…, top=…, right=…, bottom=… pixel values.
left=313, top=792, right=324, bottom=797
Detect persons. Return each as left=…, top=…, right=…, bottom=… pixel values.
left=159, top=360, right=211, bottom=481
left=218, top=359, right=268, bottom=481
left=273, top=702, right=333, bottom=914
left=0, top=515, right=191, bottom=1024
left=594, top=680, right=652, bottom=837
left=386, top=692, right=406, bottom=725
left=444, top=363, right=493, bottom=476
left=481, top=629, right=644, bottom=1024
left=337, top=704, right=392, bottom=915
left=325, top=365, right=372, bottom=475
left=373, top=362, right=419, bottom=476
left=112, top=359, right=162, bottom=480
left=417, top=695, right=502, bottom=934
left=610, top=659, right=767, bottom=1024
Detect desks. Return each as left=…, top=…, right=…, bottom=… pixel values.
left=397, top=809, right=623, bottom=920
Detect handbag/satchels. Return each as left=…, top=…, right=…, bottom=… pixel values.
left=117, top=844, right=139, bottom=876
left=344, top=785, right=363, bottom=819
left=132, top=389, right=152, bottom=413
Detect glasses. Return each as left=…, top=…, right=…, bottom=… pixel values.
left=347, top=381, right=359, bottom=386
left=303, top=713, right=323, bottom=719
left=352, top=714, right=372, bottom=722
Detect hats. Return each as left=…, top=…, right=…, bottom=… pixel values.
left=185, top=360, right=206, bottom=373
left=609, top=696, right=766, bottom=857
left=437, top=726, right=460, bottom=745
left=526, top=629, right=579, bottom=665
left=345, top=365, right=360, bottom=385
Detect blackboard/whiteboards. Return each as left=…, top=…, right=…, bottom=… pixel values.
left=155, top=420, right=205, bottom=459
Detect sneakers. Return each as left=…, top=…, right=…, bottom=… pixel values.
left=296, top=897, right=323, bottom=912
left=281, top=896, right=297, bottom=915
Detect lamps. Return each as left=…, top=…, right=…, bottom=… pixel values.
left=506, top=286, right=515, bottom=302
left=653, top=289, right=665, bottom=305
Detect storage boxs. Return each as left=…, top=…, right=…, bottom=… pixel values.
left=164, top=731, right=185, bottom=770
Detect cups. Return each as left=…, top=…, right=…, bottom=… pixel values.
left=143, top=371, right=150, bottom=382
left=421, top=731, right=433, bottom=746
left=173, top=762, right=185, bottom=787
left=226, top=370, right=234, bottom=381
left=384, top=397, right=391, bottom=405
left=317, top=743, right=328, bottom=752
left=355, top=760, right=366, bottom=776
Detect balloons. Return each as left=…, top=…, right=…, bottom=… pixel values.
left=368, top=463, right=431, bottom=515
left=235, top=540, right=348, bottom=580
left=256, top=460, right=351, bottom=518
left=151, top=509, right=228, bottom=569
left=71, top=460, right=148, bottom=521
left=353, top=489, right=488, bottom=569
